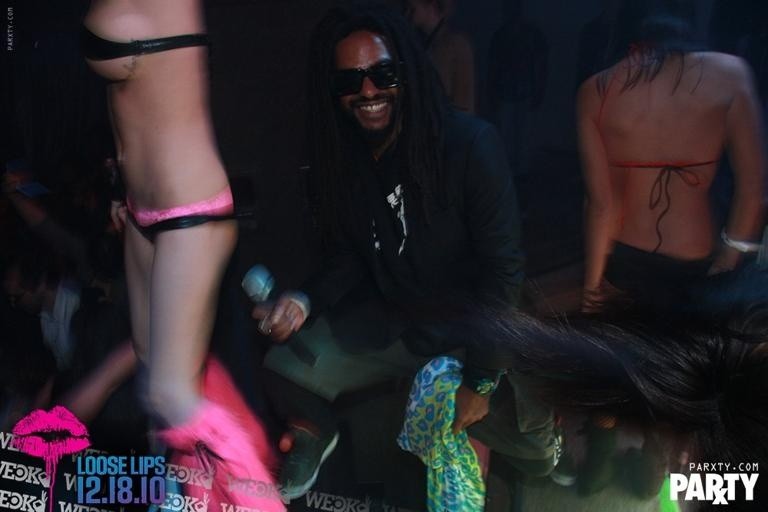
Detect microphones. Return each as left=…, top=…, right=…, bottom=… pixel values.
left=241, top=265, right=320, bottom=364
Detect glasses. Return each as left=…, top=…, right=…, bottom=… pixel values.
left=327, top=61, right=399, bottom=98
left=6, top=291, right=26, bottom=305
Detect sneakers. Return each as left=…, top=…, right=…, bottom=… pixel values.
left=550, top=427, right=579, bottom=487
left=276, top=421, right=340, bottom=501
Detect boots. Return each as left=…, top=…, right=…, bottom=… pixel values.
left=577, top=423, right=623, bottom=495
left=626, top=440, right=669, bottom=501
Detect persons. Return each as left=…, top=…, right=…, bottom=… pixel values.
left=0, top=1, right=768, bottom=512
